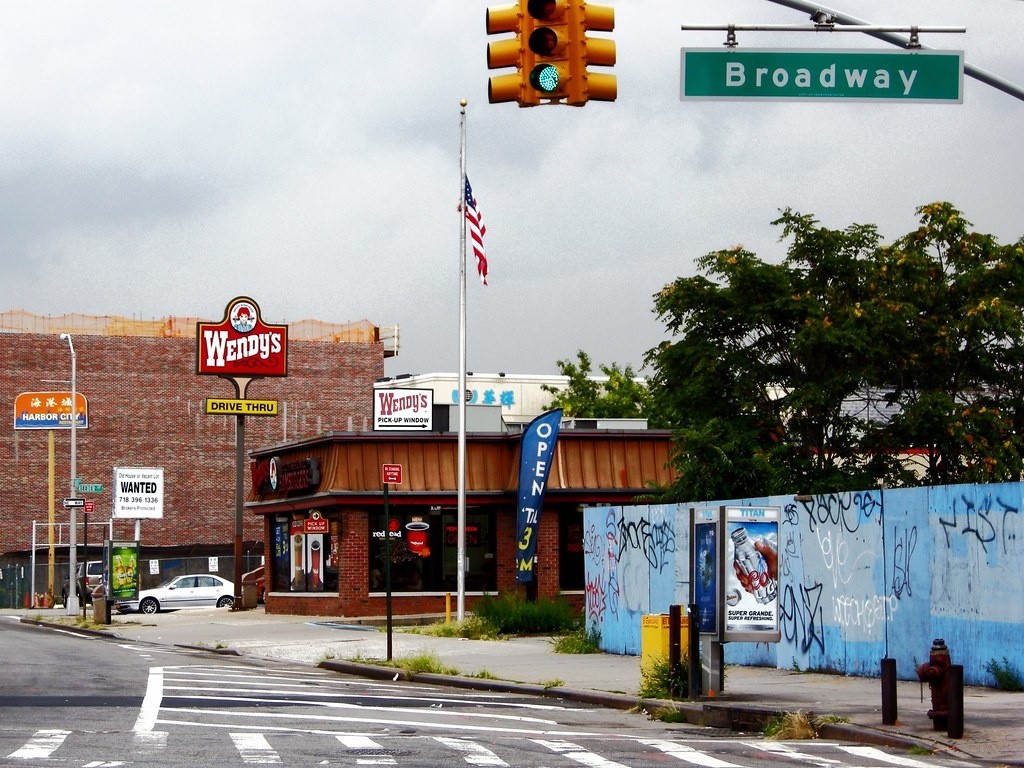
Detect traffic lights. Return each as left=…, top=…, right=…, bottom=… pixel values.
left=484, top=2, right=530, bottom=108
left=580, top=1, right=619, bottom=105
left=519, top=0, right=587, bottom=107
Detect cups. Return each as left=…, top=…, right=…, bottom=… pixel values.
left=405, top=521, right=429, bottom=553
left=293, top=531, right=303, bottom=588
left=311, top=540, right=320, bottom=585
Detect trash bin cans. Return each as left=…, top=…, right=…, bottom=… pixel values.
left=640, top=612, right=689, bottom=693
left=241, top=572, right=258, bottom=609
left=91, top=584, right=106, bottom=624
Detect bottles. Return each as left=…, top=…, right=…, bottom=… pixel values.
left=126, top=556, right=134, bottom=585
left=731, top=527, right=777, bottom=605
left=115, top=555, right=126, bottom=586
left=336, top=554, right=338, bottom=568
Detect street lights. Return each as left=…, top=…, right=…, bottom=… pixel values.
left=60, top=334, right=82, bottom=616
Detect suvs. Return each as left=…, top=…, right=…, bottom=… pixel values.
left=59, top=560, right=115, bottom=608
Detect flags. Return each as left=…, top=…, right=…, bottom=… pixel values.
left=457, top=175, right=488, bottom=285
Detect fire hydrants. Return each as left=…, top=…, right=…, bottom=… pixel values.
left=912, top=638, right=951, bottom=734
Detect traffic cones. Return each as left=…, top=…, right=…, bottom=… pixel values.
left=23, top=591, right=30, bottom=607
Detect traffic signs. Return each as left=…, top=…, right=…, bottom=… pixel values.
left=63, top=497, right=86, bottom=508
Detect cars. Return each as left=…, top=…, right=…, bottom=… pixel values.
left=242, top=561, right=266, bottom=605
left=115, top=573, right=237, bottom=616
